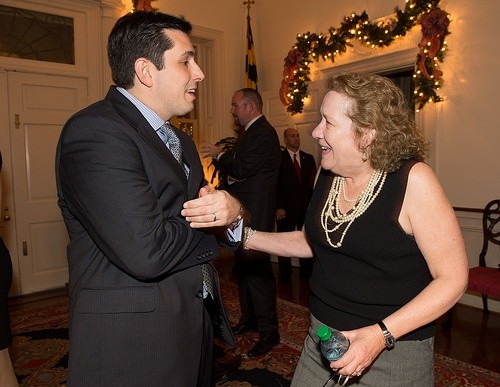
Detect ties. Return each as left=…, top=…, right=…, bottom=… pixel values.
left=233, top=129, right=246, bottom=159
left=293, top=154, right=302, bottom=183
left=159, top=123, right=215, bottom=300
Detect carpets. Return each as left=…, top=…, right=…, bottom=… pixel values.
left=9, top=276, right=500, bottom=387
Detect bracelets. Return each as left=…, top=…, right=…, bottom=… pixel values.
left=242, top=227, right=256, bottom=250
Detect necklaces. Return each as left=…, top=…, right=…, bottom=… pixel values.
left=321, top=164, right=388, bottom=247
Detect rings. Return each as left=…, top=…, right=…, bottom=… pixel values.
left=356, top=370, right=362, bottom=376
left=213, top=214, right=216, bottom=221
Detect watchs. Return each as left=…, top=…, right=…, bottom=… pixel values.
left=373, top=320, right=395, bottom=349
left=229, top=193, right=245, bottom=223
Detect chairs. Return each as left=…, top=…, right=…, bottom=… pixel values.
left=447, top=199, right=500, bottom=325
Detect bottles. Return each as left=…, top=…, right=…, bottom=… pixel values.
left=316, top=326, right=349, bottom=363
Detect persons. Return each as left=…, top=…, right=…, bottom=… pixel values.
left=251, top=70, right=470, bottom=387
left=200, top=89, right=318, bottom=360
left=55, top=10, right=251, bottom=387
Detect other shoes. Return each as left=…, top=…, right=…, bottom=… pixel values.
left=300, top=263, right=313, bottom=279
left=281, top=271, right=292, bottom=284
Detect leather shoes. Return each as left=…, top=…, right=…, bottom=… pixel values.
left=232, top=318, right=259, bottom=336
left=246, top=335, right=281, bottom=357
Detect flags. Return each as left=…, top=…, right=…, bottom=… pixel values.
left=244, top=9, right=259, bottom=91
